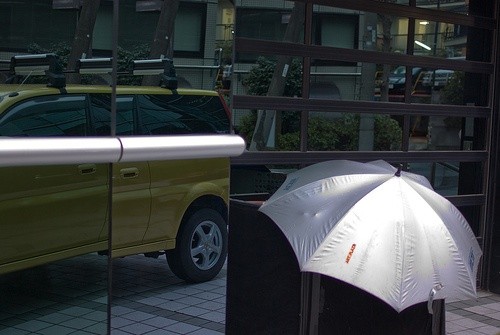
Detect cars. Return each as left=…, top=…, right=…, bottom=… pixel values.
left=424, top=56, right=466, bottom=89
left=381, top=65, right=423, bottom=93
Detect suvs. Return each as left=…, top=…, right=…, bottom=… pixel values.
left=1, top=53, right=245, bottom=284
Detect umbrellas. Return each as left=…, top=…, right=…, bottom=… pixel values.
left=257, top=160, right=484, bottom=313
